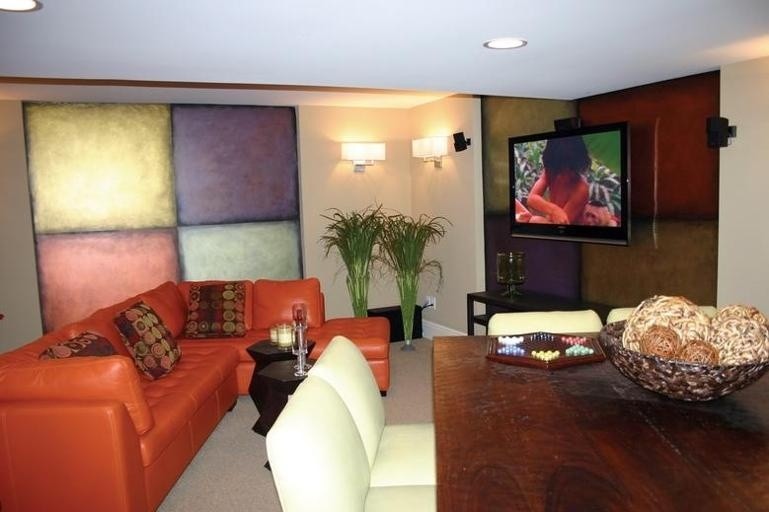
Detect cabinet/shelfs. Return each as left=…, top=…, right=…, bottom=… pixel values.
left=467, top=289, right=617, bottom=337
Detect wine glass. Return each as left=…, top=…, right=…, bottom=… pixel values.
left=291, top=326, right=312, bottom=376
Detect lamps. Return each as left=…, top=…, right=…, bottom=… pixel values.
left=341, top=142, right=386, bottom=173
left=412, top=137, right=447, bottom=168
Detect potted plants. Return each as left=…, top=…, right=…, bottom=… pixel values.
left=317, top=200, right=452, bottom=351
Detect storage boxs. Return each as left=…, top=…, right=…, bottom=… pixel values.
left=367, top=304, right=422, bottom=341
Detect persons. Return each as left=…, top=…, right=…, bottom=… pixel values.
left=526, top=138, right=590, bottom=227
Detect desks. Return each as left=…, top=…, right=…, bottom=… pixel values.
left=433, top=336, right=769, bottom=512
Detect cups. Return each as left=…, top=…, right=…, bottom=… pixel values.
left=270, top=329, right=277, bottom=345
left=276, top=326, right=293, bottom=353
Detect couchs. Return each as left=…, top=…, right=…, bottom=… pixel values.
left=0, top=263, right=389, bottom=512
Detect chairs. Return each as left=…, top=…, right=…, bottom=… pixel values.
left=606, top=308, right=633, bottom=324
left=309, top=337, right=438, bottom=485
left=487, top=309, right=602, bottom=338
left=265, top=376, right=436, bottom=511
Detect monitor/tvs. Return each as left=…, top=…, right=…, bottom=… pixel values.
left=507, top=119, right=633, bottom=248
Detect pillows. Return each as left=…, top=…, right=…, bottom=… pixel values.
left=185, top=284, right=245, bottom=337
left=113, top=300, right=181, bottom=380
left=40, top=330, right=115, bottom=360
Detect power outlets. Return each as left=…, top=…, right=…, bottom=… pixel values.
left=423, top=296, right=429, bottom=309
left=429, top=296, right=436, bottom=309
